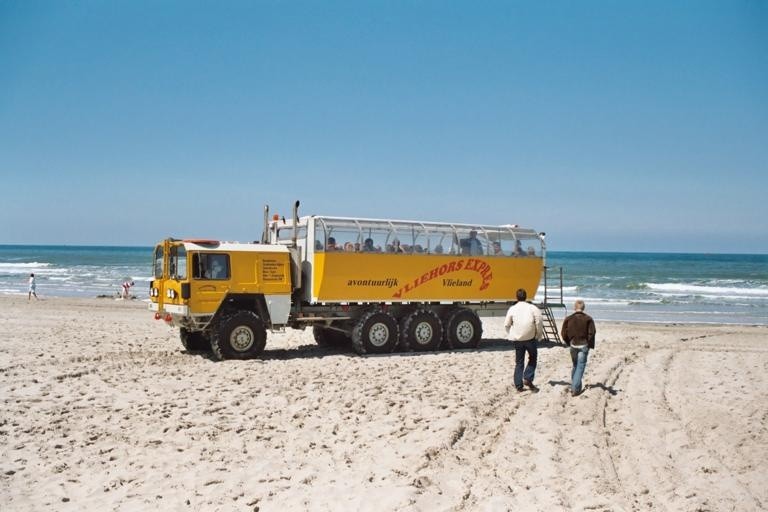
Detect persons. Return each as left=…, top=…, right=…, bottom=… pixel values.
left=504, top=287, right=544, bottom=391
left=560, top=300, right=596, bottom=396
left=122, top=281, right=135, bottom=299
left=202, top=255, right=222, bottom=279
left=315, top=227, right=536, bottom=257
left=28, top=273, right=38, bottom=300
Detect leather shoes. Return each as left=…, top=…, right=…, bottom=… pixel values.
left=523, top=379, right=534, bottom=388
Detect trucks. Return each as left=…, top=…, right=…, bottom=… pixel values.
left=150, top=214, right=548, bottom=361
